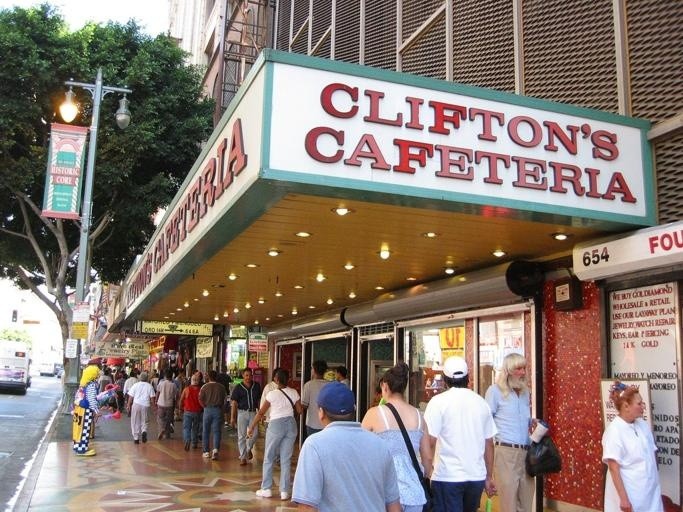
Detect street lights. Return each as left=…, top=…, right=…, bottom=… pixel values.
left=54, top=65, right=136, bottom=440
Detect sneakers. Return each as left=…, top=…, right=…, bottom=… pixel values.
left=191, top=444, right=198, bottom=449
left=133, top=439, right=140, bottom=444
left=183, top=440, right=191, bottom=452
left=210, top=449, right=218, bottom=461
left=157, top=424, right=176, bottom=440
left=239, top=458, right=247, bottom=466
left=75, top=447, right=96, bottom=457
left=201, top=451, right=210, bottom=458
left=280, top=490, right=289, bottom=501
left=141, top=432, right=148, bottom=443
left=254, top=487, right=272, bottom=499
left=247, top=451, right=254, bottom=461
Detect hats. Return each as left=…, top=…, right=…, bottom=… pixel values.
left=314, top=380, right=355, bottom=416
left=442, top=354, right=470, bottom=380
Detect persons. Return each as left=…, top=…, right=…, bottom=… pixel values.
left=482, top=350, right=541, bottom=512
left=96, top=360, right=353, bottom=467
left=289, top=379, right=406, bottom=512
left=598, top=378, right=668, bottom=511
left=358, top=358, right=434, bottom=511
left=419, top=355, right=499, bottom=511
left=69, top=364, right=122, bottom=457
left=244, top=366, right=304, bottom=501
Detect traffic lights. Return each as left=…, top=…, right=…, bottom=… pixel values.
left=11, top=309, right=19, bottom=324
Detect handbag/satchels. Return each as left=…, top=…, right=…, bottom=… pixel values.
left=292, top=406, right=300, bottom=421
left=419, top=476, right=436, bottom=512
left=523, top=434, right=565, bottom=475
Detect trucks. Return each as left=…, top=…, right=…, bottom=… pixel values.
left=0, top=339, right=33, bottom=395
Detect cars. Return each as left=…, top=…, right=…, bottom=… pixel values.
left=36, top=360, right=67, bottom=381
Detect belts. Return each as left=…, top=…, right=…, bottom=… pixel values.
left=241, top=409, right=257, bottom=413
left=492, top=439, right=529, bottom=450
